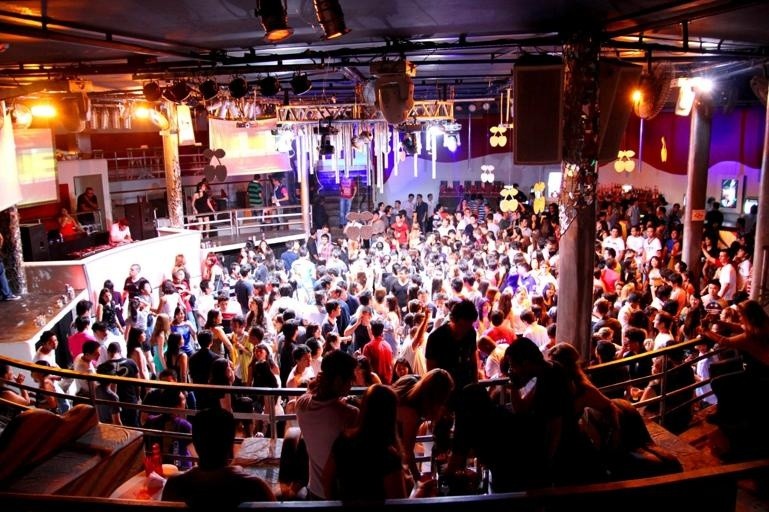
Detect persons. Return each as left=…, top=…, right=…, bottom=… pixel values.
left=0, top=168, right=768, bottom=510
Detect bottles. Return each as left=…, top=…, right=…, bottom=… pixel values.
left=143, top=442, right=164, bottom=479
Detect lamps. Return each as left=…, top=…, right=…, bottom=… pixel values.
left=488, top=123, right=508, bottom=148
left=140, top=81, right=163, bottom=102
left=270, top=120, right=464, bottom=193
left=199, top=79, right=220, bottom=101
left=533, top=164, right=546, bottom=215
left=613, top=128, right=636, bottom=173
left=498, top=185, right=520, bottom=213
left=85, top=106, right=99, bottom=130
left=101, top=105, right=112, bottom=131
left=110, top=103, right=120, bottom=130
left=242, top=100, right=259, bottom=120
left=600, top=39, right=717, bottom=121
left=204, top=99, right=223, bottom=112
left=252, top=0, right=355, bottom=44
left=290, top=73, right=315, bottom=98
left=228, top=78, right=253, bottom=99
left=227, top=98, right=240, bottom=121
left=122, top=101, right=134, bottom=130
left=479, top=164, right=497, bottom=183
left=169, top=81, right=195, bottom=103
left=258, top=75, right=283, bottom=99
left=218, top=99, right=231, bottom=120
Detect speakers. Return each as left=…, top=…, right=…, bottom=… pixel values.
left=19, top=224, right=51, bottom=261
left=598, top=57, right=643, bottom=166
left=512, top=55, right=564, bottom=165
left=125, top=203, right=158, bottom=240
